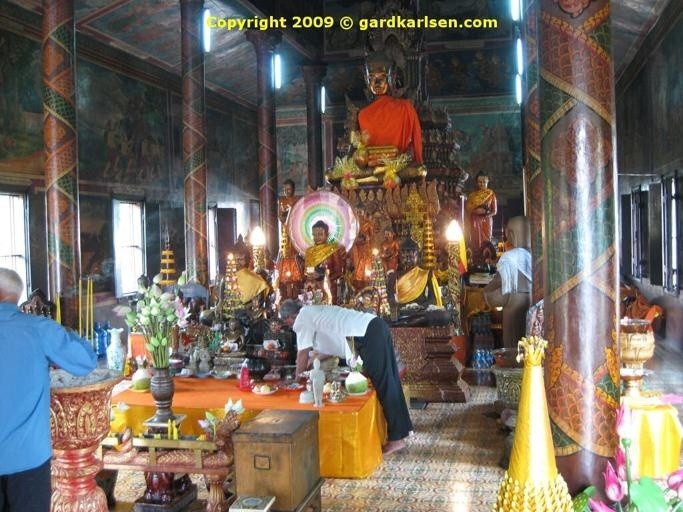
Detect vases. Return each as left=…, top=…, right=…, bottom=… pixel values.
left=150, top=367, right=176, bottom=422
left=222, top=491, right=237, bottom=506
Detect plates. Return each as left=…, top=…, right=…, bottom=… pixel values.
left=252, top=389, right=277, bottom=395
left=130, top=387, right=150, bottom=393
left=343, top=390, right=367, bottom=396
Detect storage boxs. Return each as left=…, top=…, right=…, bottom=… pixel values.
left=230, top=410, right=320, bottom=512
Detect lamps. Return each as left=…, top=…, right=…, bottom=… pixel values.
left=516, top=36, right=524, bottom=75
left=320, top=84, right=326, bottom=115
left=271, top=53, right=282, bottom=90
left=203, top=7, right=211, bottom=54
left=509, top=0, right=525, bottom=28
left=445, top=219, right=465, bottom=337
left=514, top=74, right=523, bottom=107
left=250, top=225, right=266, bottom=269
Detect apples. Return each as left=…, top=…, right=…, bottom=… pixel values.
left=260, top=383, right=271, bottom=393
left=254, top=384, right=260, bottom=392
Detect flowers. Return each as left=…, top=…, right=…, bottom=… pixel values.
left=111, top=273, right=192, bottom=368
left=197, top=396, right=246, bottom=494
left=587, top=400, right=683, bottom=512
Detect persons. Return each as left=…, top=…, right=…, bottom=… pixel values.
left=351, top=205, right=371, bottom=265
left=342, top=62, right=419, bottom=158
left=301, top=224, right=349, bottom=298
left=465, top=170, right=500, bottom=252
left=388, top=228, right=452, bottom=328
left=376, top=227, right=402, bottom=292
left=428, top=250, right=461, bottom=305
left=1, top=266, right=98, bottom=511
left=485, top=216, right=532, bottom=359
left=224, top=317, right=246, bottom=351
left=309, top=357, right=329, bottom=407
left=269, top=180, right=303, bottom=226
left=262, top=317, right=286, bottom=350
left=221, top=234, right=265, bottom=311
left=269, top=299, right=417, bottom=456
